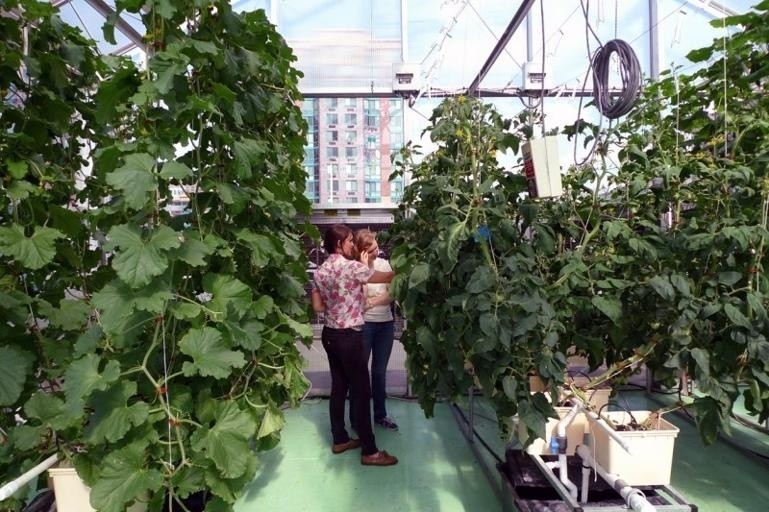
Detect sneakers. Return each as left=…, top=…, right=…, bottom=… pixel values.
left=378, top=419, right=398, bottom=431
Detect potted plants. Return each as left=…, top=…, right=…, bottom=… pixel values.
left=383, top=95, right=769, bottom=488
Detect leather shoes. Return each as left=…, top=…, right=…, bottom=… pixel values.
left=361, top=451, right=398, bottom=466
left=332, top=439, right=361, bottom=453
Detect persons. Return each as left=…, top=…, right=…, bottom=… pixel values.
left=312, top=224, right=396, bottom=466
